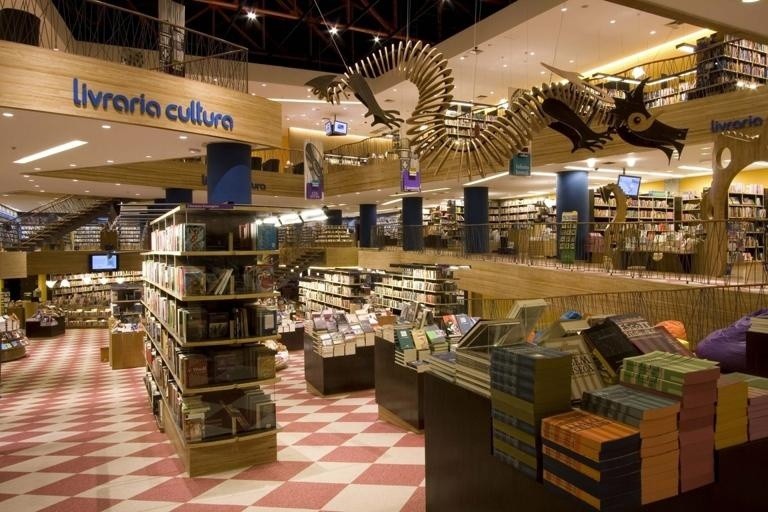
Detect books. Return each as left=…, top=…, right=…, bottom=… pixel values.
left=728, top=195, right=763, bottom=205
left=111, top=319, right=143, bottom=332
left=242, top=262, right=274, bottom=293
left=488, top=206, right=548, bottom=213
left=543, top=335, right=604, bottom=403
left=304, top=306, right=398, bottom=359
left=0, top=291, right=13, bottom=317
left=277, top=225, right=296, bottom=243
left=422, top=199, right=464, bottom=240
left=489, top=223, right=534, bottom=228
left=593, top=195, right=673, bottom=208
left=251, top=304, right=279, bottom=336
left=373, top=285, right=454, bottom=304
left=307, top=300, right=332, bottom=312
left=141, top=259, right=207, bottom=297
left=714, top=376, right=750, bottom=452
left=206, top=268, right=237, bottom=296
left=276, top=302, right=304, bottom=334
left=299, top=287, right=351, bottom=308
left=301, top=221, right=322, bottom=242
left=143, top=370, right=167, bottom=433
left=619, top=350, right=721, bottom=494
left=316, top=225, right=353, bottom=243
left=150, top=223, right=207, bottom=251
left=381, top=276, right=444, bottom=290
left=727, top=183, right=765, bottom=194
left=593, top=207, right=673, bottom=218
left=442, top=101, right=501, bottom=142
left=375, top=302, right=490, bottom=398
left=728, top=206, right=767, bottom=219
left=623, top=224, right=703, bottom=254
left=26, top=304, right=66, bottom=322
left=488, top=300, right=617, bottom=319
left=244, top=343, right=276, bottom=380
left=606, top=309, right=696, bottom=358
left=539, top=406, right=641, bottom=511
left=406, top=267, right=443, bottom=279
left=210, top=349, right=244, bottom=383
left=142, top=306, right=210, bottom=389
left=298, top=278, right=359, bottom=296
left=241, top=386, right=277, bottom=429
left=488, top=197, right=556, bottom=207
left=488, top=213, right=546, bottom=221
left=726, top=251, right=756, bottom=263
left=0, top=204, right=144, bottom=250
left=682, top=193, right=704, bottom=227
left=376, top=212, right=404, bottom=246
left=489, top=343, right=574, bottom=479
left=582, top=319, right=643, bottom=384
left=0, top=312, right=30, bottom=351
left=729, top=220, right=768, bottom=234
left=609, top=35, right=767, bottom=108
left=44, top=271, right=144, bottom=329
left=323, top=273, right=355, bottom=285
left=208, top=307, right=229, bottom=340
left=457, top=317, right=519, bottom=350
left=229, top=306, right=250, bottom=339
left=143, top=283, right=206, bottom=342
left=732, top=371, right=767, bottom=441
left=581, top=382, right=681, bottom=507
left=144, top=336, right=210, bottom=444
left=746, top=315, right=768, bottom=332
left=729, top=234, right=761, bottom=248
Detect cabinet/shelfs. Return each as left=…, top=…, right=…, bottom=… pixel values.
left=280, top=327, right=306, bottom=350
left=374, top=335, right=423, bottom=435
left=296, top=267, right=382, bottom=314
left=26, top=314, right=66, bottom=340
left=587, top=187, right=672, bottom=248
left=139, top=203, right=282, bottom=480
left=672, top=196, right=702, bottom=253
left=694, top=31, right=768, bottom=90
left=51, top=271, right=141, bottom=330
left=726, top=188, right=768, bottom=284
left=424, top=369, right=721, bottom=511
left=422, top=205, right=448, bottom=234
left=595, top=78, right=697, bottom=111
left=447, top=200, right=499, bottom=248
left=499, top=192, right=555, bottom=239
left=371, top=263, right=468, bottom=324
left=0, top=311, right=28, bottom=362
left=303, top=332, right=376, bottom=398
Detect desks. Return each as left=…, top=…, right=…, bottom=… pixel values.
left=109, top=322, right=147, bottom=370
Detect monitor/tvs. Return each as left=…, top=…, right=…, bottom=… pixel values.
left=324, top=121, right=333, bottom=135
left=616, top=174, right=641, bottom=201
left=333, top=120, right=348, bottom=135
left=88, top=253, right=120, bottom=273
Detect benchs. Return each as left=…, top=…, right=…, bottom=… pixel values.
left=618, top=249, right=695, bottom=274
left=685, top=81, right=737, bottom=101
